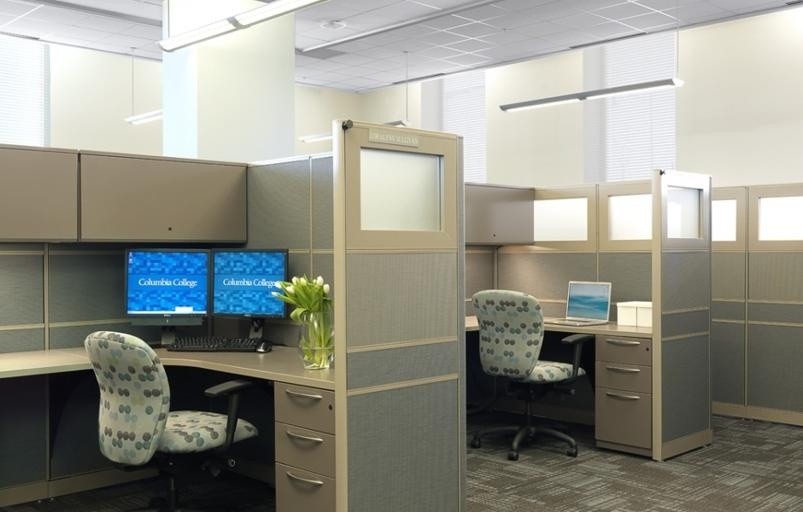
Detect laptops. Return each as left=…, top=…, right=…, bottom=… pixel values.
left=544, top=280, right=612, bottom=326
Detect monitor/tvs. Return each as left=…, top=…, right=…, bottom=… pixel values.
left=122, top=247, right=212, bottom=348
left=212, top=245, right=290, bottom=342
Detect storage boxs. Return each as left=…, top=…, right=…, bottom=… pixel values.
left=617, top=300, right=653, bottom=328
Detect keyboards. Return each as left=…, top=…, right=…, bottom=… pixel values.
left=165, top=336, right=261, bottom=353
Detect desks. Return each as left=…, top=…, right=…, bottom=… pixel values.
left=465, top=315, right=652, bottom=460
left=0, top=345, right=335, bottom=512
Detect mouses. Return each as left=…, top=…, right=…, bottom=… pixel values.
left=257, top=340, right=272, bottom=353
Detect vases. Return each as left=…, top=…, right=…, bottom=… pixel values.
left=298, top=311, right=335, bottom=369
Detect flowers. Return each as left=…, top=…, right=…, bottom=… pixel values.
left=272, top=274, right=334, bottom=367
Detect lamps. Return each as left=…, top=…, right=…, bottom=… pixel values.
left=124, top=46, right=162, bottom=125
left=300, top=51, right=409, bottom=144
left=499, top=0, right=683, bottom=113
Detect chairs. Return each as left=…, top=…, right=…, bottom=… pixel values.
left=472, top=290, right=594, bottom=461
left=84, top=331, right=259, bottom=512
